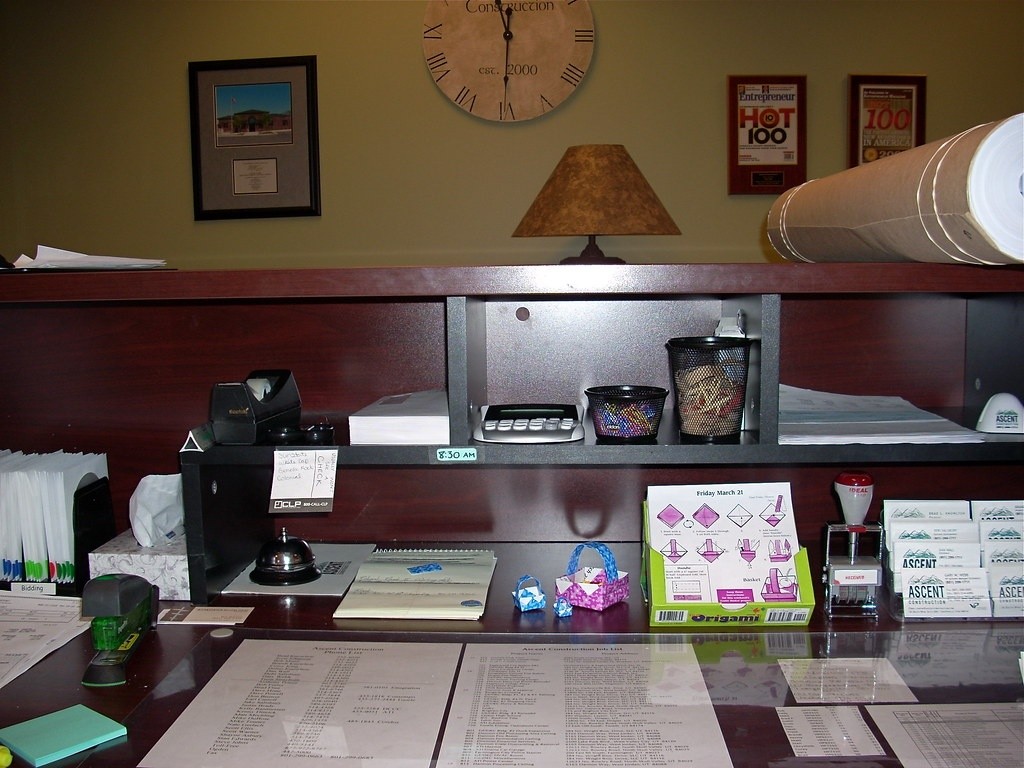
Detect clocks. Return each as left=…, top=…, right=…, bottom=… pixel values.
left=422, top=0, right=593, bottom=121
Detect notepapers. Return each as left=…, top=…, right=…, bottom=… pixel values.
left=0, top=701, right=128, bottom=768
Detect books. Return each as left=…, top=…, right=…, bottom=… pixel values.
left=0, top=448, right=110, bottom=583
left=348, top=389, right=451, bottom=446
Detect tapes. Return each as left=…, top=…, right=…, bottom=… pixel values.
left=245, top=378, right=271, bottom=403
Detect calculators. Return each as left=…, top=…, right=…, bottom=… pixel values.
left=472, top=403, right=585, bottom=445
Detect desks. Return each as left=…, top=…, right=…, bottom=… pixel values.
left=0, top=541, right=1024, bottom=767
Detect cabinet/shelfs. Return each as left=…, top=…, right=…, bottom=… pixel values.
left=2, top=260, right=1023, bottom=632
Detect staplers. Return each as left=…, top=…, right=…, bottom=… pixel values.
left=79, top=573, right=159, bottom=687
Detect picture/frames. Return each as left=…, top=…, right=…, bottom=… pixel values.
left=189, top=55, right=322, bottom=219
left=726, top=74, right=807, bottom=195
left=847, top=72, right=927, bottom=170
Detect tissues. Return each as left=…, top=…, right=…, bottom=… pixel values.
left=87, top=473, right=191, bottom=601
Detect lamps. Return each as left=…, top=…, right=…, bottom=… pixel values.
left=512, top=141, right=681, bottom=266
